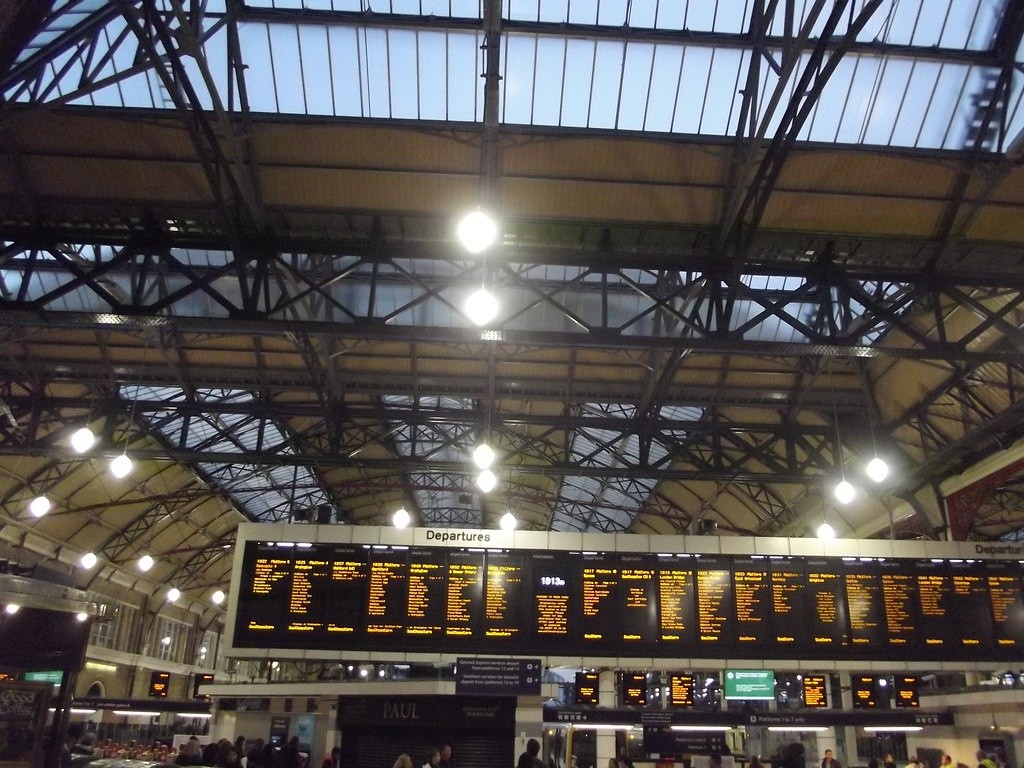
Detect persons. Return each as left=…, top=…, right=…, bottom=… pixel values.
left=422, top=744, right=452, bottom=768
left=749, top=754, right=764, bottom=768
left=517, top=739, right=547, bottom=768
left=175, top=735, right=303, bottom=768
left=74, top=732, right=96, bottom=754
left=867, top=750, right=1009, bottom=768
left=393, top=754, right=412, bottom=768
left=609, top=756, right=636, bottom=768
left=821, top=749, right=840, bottom=767
left=322, top=746, right=342, bottom=768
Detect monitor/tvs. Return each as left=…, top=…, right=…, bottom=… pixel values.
left=724, top=668, right=775, bottom=700
left=24, top=671, right=63, bottom=687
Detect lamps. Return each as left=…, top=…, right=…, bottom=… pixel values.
left=81, top=522, right=97, bottom=568
left=138, top=520, right=154, bottom=570
left=814, top=478, right=835, bottom=542
left=827, top=360, right=854, bottom=506
left=465, top=251, right=499, bottom=327
left=30, top=455, right=57, bottom=517
left=458, top=74, right=496, bottom=254
left=861, top=389, right=888, bottom=482
left=111, top=328, right=147, bottom=480
left=477, top=469, right=496, bottom=493
left=212, top=553, right=225, bottom=603
left=167, top=568, right=184, bottom=601
left=392, top=472, right=411, bottom=529
left=472, top=340, right=494, bottom=469
left=499, top=469, right=518, bottom=531
left=72, top=326, right=110, bottom=454
